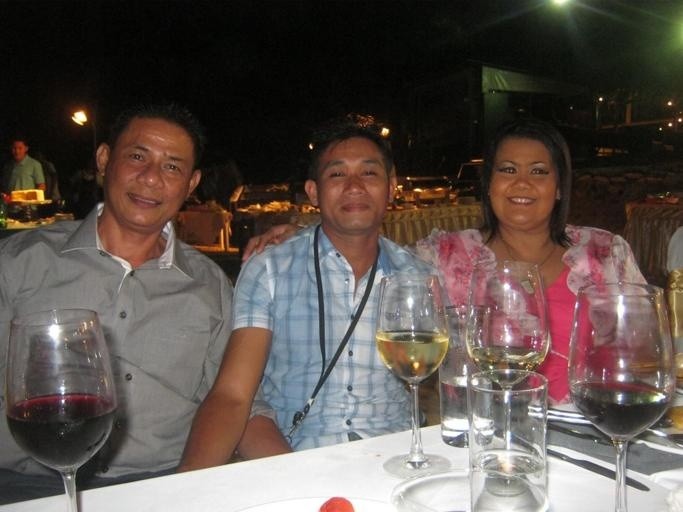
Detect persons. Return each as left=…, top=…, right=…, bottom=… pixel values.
left=179, top=124, right=461, bottom=476
left=6, top=141, right=47, bottom=202
left=242, top=120, right=652, bottom=407
left=0, top=100, right=296, bottom=506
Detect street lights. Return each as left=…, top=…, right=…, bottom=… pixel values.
left=71, top=108, right=99, bottom=205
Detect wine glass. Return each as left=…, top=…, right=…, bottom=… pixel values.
left=374, top=272, right=453, bottom=482
left=567, top=280, right=677, bottom=512
left=463, top=259, right=552, bottom=482
left=4, top=308, right=120, bottom=512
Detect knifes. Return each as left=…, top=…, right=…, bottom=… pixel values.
left=547, top=447, right=650, bottom=492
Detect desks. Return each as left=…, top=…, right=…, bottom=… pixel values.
left=1, top=404, right=683, bottom=512
left=625, top=201, right=683, bottom=277
left=258, top=203, right=484, bottom=244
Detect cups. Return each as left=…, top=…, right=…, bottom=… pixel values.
left=436, top=305, right=497, bottom=448
left=466, top=367, right=549, bottom=512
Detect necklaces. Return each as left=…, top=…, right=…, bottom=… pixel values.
left=497, top=230, right=555, bottom=295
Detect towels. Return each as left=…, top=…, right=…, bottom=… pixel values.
left=549, top=421, right=683, bottom=476
